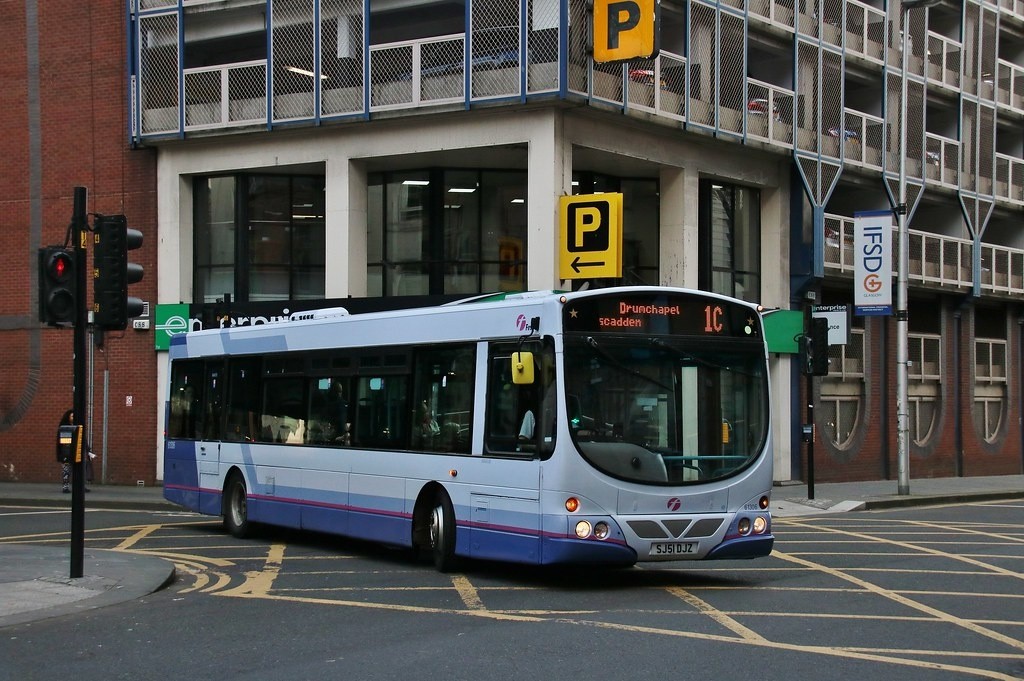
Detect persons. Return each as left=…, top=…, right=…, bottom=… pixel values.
left=57, top=410, right=91, bottom=494
left=320, top=399, right=598, bottom=452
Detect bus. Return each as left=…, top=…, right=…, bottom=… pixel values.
left=165, top=284, right=782, bottom=573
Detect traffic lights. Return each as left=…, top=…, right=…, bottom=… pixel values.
left=37, top=246, right=79, bottom=323
left=96, top=213, right=143, bottom=329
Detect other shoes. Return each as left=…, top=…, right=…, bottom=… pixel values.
left=63, top=488, right=71, bottom=493
